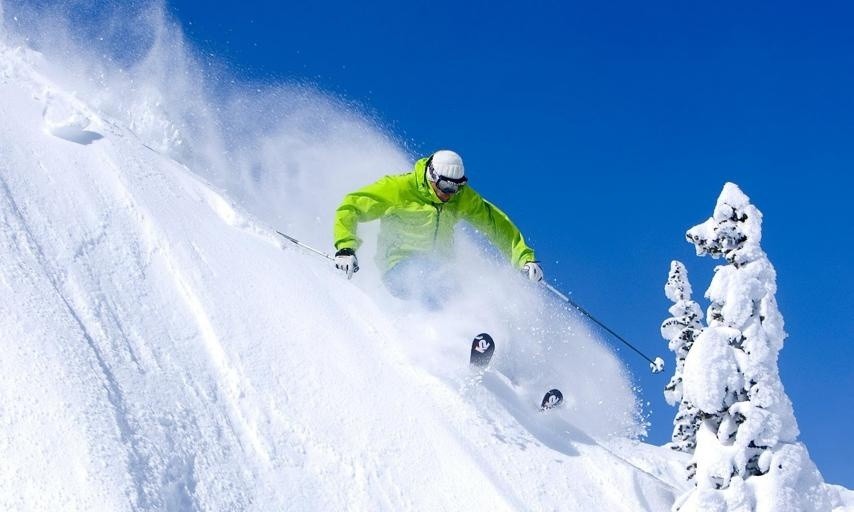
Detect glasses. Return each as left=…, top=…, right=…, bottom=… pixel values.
left=428, top=164, right=467, bottom=194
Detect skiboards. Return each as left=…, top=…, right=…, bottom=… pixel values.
left=470, top=333, right=563, bottom=412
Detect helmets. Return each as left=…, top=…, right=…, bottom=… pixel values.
left=427, top=150, right=464, bottom=185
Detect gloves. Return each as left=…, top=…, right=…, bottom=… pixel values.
left=336, top=248, right=358, bottom=281
left=521, top=261, right=544, bottom=284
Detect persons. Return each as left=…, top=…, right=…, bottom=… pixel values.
left=332, top=149, right=543, bottom=316
